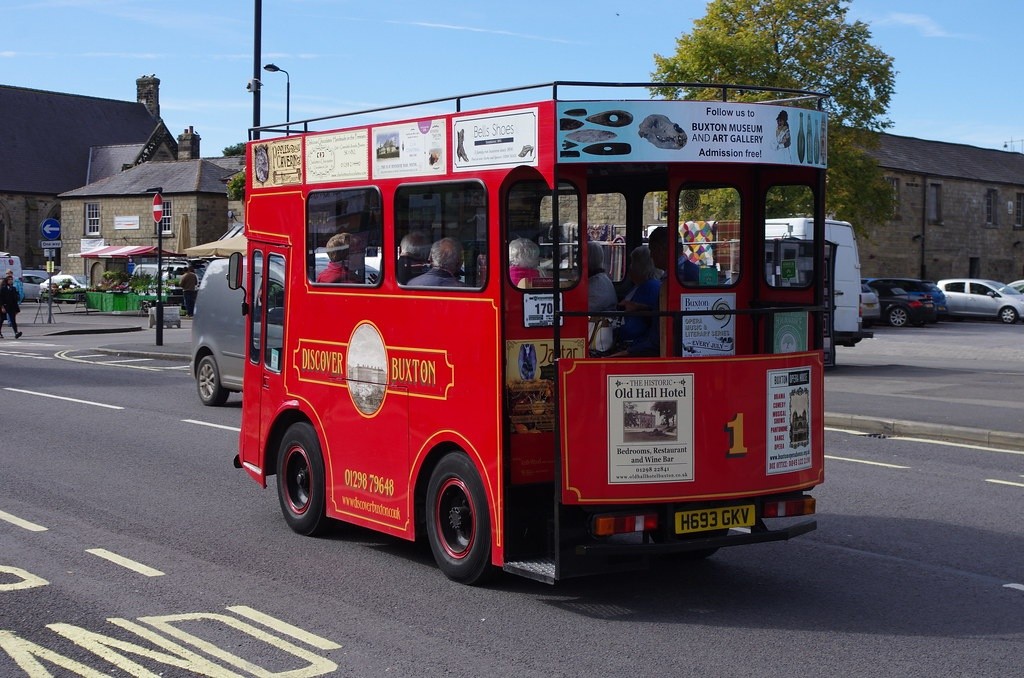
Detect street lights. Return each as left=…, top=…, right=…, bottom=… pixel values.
left=263, top=63, right=290, bottom=136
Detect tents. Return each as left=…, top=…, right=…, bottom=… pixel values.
left=66, top=245, right=186, bottom=275
left=183, top=234, right=247, bottom=257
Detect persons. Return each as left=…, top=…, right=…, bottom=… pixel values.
left=0, top=269, right=24, bottom=340
left=317, top=225, right=700, bottom=360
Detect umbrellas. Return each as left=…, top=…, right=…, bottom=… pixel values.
left=175, top=212, right=191, bottom=254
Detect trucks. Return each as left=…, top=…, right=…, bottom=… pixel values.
left=0, top=252, right=24, bottom=304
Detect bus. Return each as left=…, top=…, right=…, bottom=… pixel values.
left=232, top=81, right=828, bottom=589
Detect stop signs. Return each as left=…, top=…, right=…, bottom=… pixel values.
left=152, top=194, right=164, bottom=224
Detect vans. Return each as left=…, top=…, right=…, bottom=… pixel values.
left=641, top=218, right=874, bottom=348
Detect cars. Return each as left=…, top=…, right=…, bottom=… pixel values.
left=865, top=278, right=937, bottom=326
left=861, top=283, right=882, bottom=329
left=993, top=280, right=1024, bottom=294
left=131, top=263, right=205, bottom=289
left=21, top=274, right=46, bottom=303
left=936, top=278, right=1024, bottom=324
left=39, top=275, right=90, bottom=304
left=22, top=270, right=49, bottom=281
left=189, top=252, right=380, bottom=406
left=859, top=278, right=949, bottom=320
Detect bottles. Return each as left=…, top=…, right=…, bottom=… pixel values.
left=797, top=112, right=827, bottom=166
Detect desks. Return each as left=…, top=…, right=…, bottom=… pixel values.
left=41, top=291, right=88, bottom=315
left=86, top=292, right=138, bottom=311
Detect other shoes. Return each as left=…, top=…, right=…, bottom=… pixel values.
left=0, top=334, right=3, bottom=338
left=15, top=332, right=22, bottom=339
